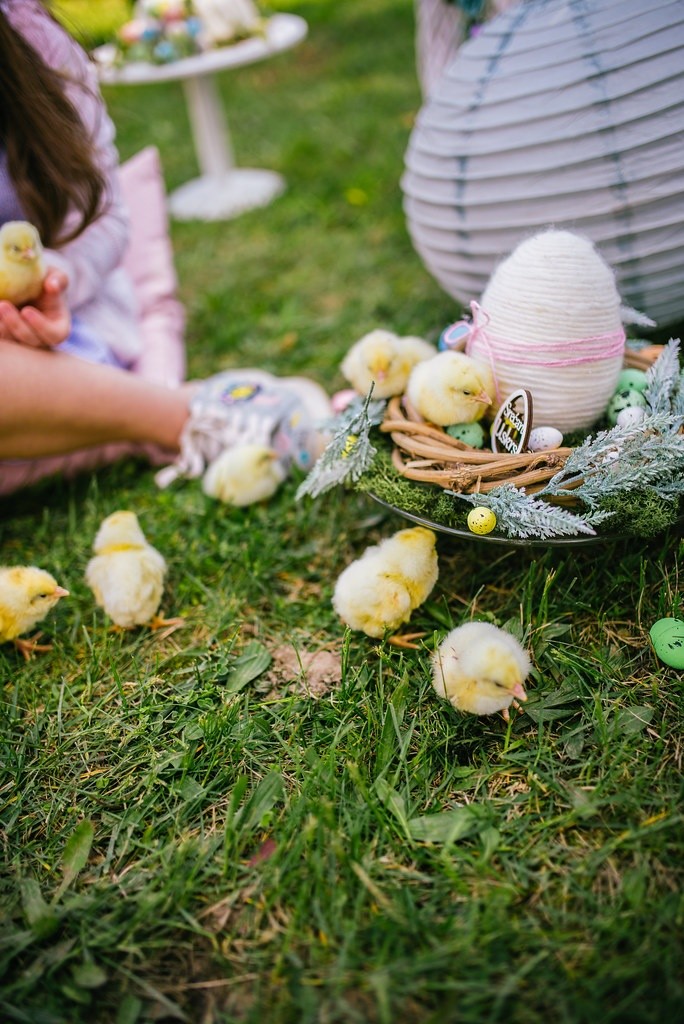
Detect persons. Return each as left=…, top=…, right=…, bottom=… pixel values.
left=0, top=0, right=343, bottom=496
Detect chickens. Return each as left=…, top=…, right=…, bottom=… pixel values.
left=337, top=329, right=438, bottom=401
left=0, top=220, right=47, bottom=308
left=0, top=566, right=69, bottom=663
left=432, top=621, right=533, bottom=726
left=202, top=444, right=286, bottom=510
left=82, top=509, right=183, bottom=636
left=408, top=351, right=495, bottom=427
left=329, top=527, right=440, bottom=649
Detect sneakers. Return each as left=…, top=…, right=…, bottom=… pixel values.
left=153, top=364, right=339, bottom=490
